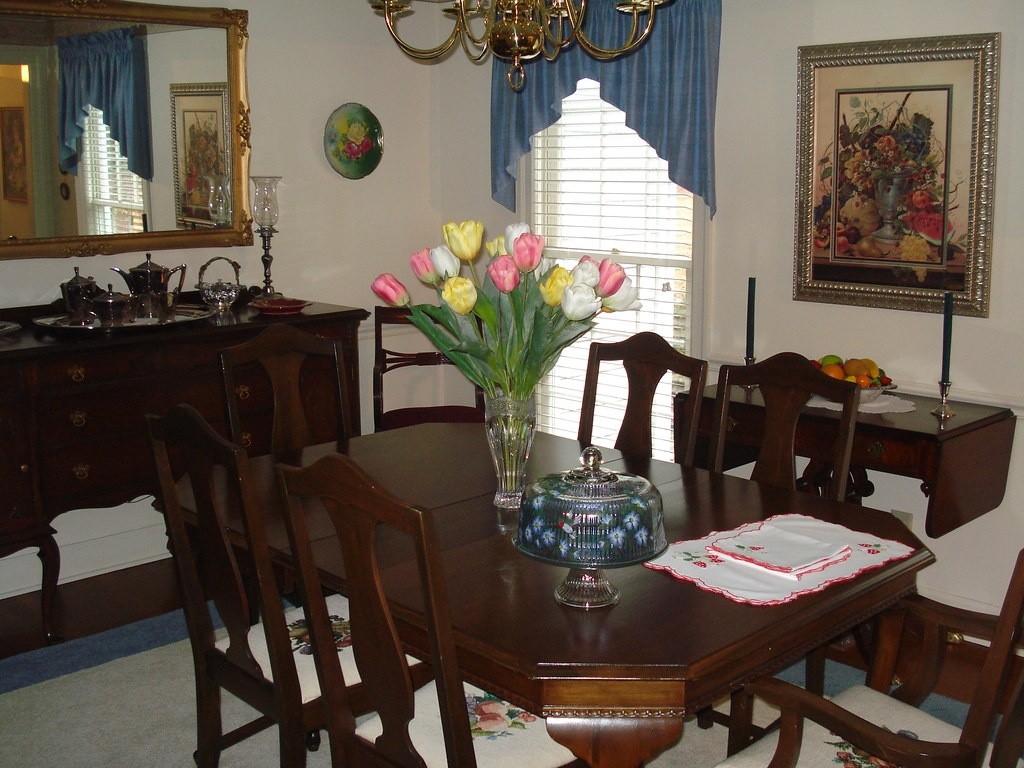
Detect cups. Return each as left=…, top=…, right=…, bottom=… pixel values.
left=59, top=267, right=96, bottom=326
left=148, top=291, right=177, bottom=323
left=94, top=284, right=139, bottom=326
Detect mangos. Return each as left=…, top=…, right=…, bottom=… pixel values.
left=859, top=359, right=879, bottom=377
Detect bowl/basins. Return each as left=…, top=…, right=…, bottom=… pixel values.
left=859, top=382, right=897, bottom=404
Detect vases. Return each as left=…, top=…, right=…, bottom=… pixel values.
left=484, top=387, right=537, bottom=509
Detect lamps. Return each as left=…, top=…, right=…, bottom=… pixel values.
left=370, top=0, right=670, bottom=92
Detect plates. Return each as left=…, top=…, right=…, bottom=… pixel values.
left=248, top=299, right=313, bottom=314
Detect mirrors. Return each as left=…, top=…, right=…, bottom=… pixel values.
left=0, top=0, right=255, bottom=260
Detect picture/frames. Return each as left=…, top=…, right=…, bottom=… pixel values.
left=793, top=32, right=1001, bottom=318
left=0, top=107, right=28, bottom=203
left=169, top=82, right=232, bottom=230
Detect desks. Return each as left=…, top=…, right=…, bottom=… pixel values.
left=674, top=384, right=1017, bottom=539
left=173, top=421, right=936, bottom=768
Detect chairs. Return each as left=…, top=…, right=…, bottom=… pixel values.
left=271, top=452, right=588, bottom=768
left=145, top=404, right=424, bottom=768
left=372, top=305, right=485, bottom=432
left=713, top=546, right=1024, bottom=768
left=577, top=331, right=708, bottom=466
left=707, top=352, right=860, bottom=503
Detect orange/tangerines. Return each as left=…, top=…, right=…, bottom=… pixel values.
left=819, top=364, right=845, bottom=381
left=855, top=375, right=869, bottom=389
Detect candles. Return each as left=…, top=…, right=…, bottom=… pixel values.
left=942, top=293, right=953, bottom=383
left=746, top=277, right=756, bottom=358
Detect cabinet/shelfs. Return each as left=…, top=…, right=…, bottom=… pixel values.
left=0, top=291, right=371, bottom=646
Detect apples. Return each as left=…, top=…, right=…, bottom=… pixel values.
left=820, top=354, right=845, bottom=366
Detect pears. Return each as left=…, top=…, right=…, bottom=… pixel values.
left=843, top=358, right=875, bottom=379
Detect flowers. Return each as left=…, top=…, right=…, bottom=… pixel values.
left=371, top=221, right=644, bottom=402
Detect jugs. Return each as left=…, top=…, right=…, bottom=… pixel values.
left=109, top=253, right=187, bottom=318
left=194, top=257, right=241, bottom=311
